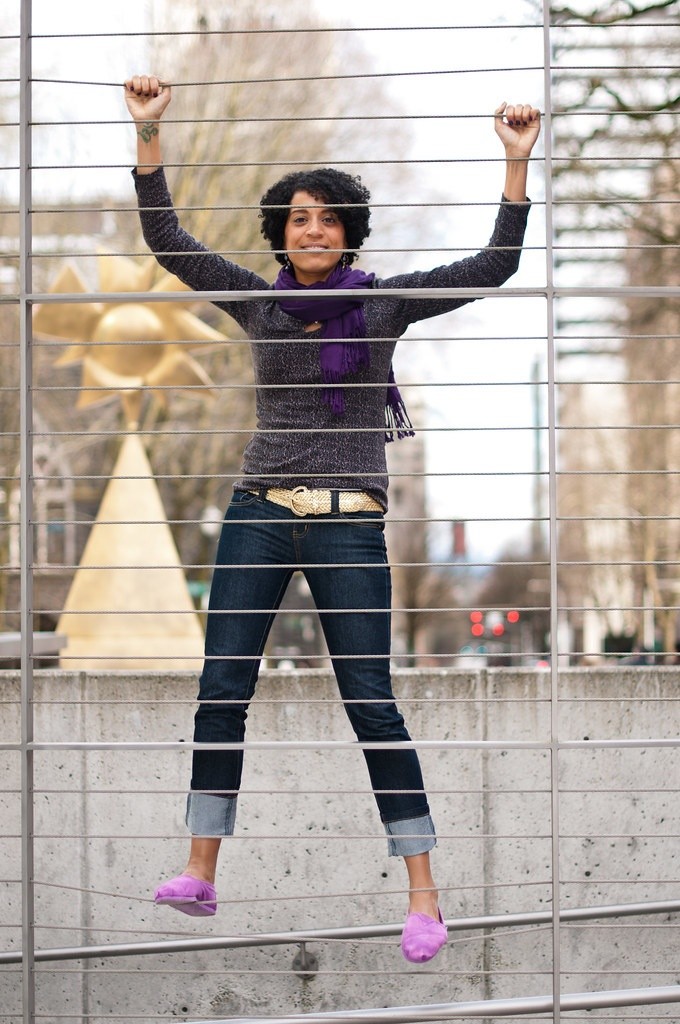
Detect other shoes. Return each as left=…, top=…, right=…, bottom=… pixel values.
left=401, top=905, right=448, bottom=963
left=155, top=874, right=217, bottom=917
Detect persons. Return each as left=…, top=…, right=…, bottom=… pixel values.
left=125, top=76, right=541, bottom=962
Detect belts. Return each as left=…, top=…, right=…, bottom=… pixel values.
left=250, top=486, right=385, bottom=517
